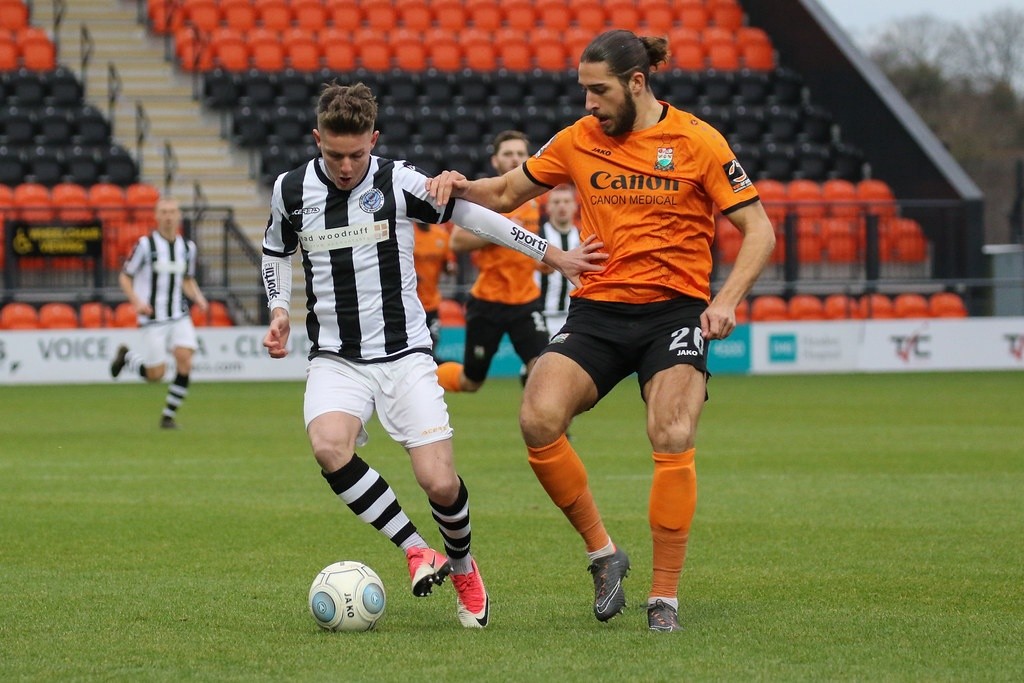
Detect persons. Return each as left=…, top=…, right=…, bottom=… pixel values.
left=261, top=82, right=609, bottom=629
left=410, top=220, right=456, bottom=358
left=425, top=29, right=775, bottom=633
left=437, top=129, right=569, bottom=438
left=533, top=182, right=582, bottom=337
left=112, top=196, right=208, bottom=432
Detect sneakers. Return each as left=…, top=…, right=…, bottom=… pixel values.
left=159, top=414, right=176, bottom=428
left=647, top=598, right=681, bottom=632
left=407, top=546, right=456, bottom=597
left=442, top=552, right=490, bottom=628
left=586, top=544, right=631, bottom=624
left=111, top=346, right=129, bottom=377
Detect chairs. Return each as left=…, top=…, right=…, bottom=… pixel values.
left=0, top=0, right=233, bottom=331
left=146, top=0, right=971, bottom=325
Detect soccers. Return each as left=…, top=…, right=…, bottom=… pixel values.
left=308, top=561, right=387, bottom=632
